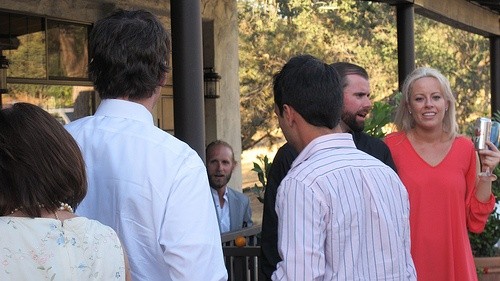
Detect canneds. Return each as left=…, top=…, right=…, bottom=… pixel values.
left=474, top=118, right=491, bottom=150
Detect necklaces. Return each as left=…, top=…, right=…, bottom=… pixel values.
left=5, top=200, right=75, bottom=220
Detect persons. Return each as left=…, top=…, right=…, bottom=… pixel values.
left=206, top=140, right=254, bottom=271
left=383, top=68, right=500, bottom=281
left=62, top=9, right=230, bottom=281
left=270, top=55, right=418, bottom=281
left=0, top=103, right=133, bottom=280
left=260, top=63, right=397, bottom=281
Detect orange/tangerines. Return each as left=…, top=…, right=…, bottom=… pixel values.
left=235, top=235, right=246, bottom=247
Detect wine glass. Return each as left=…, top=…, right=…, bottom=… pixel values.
left=478, top=121, right=500, bottom=181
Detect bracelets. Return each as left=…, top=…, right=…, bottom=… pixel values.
left=474, top=167, right=495, bottom=180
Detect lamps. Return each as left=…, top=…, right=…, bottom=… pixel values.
left=0, top=55, right=10, bottom=94
left=203, top=67, right=221, bottom=99
